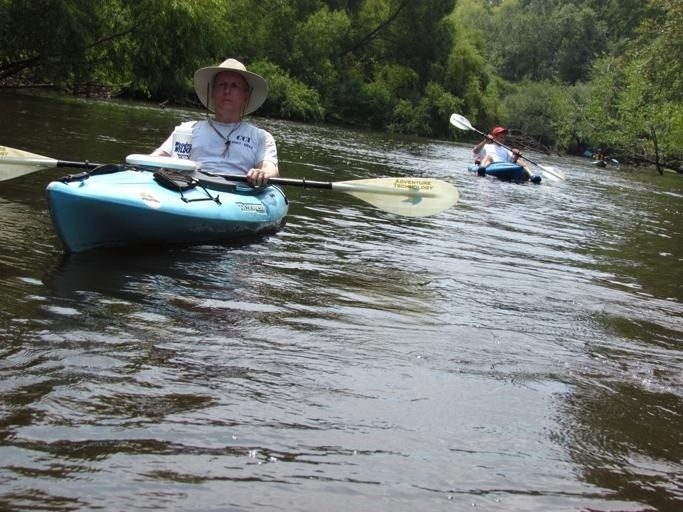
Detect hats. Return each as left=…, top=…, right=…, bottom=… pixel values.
left=492, top=127, right=508, bottom=136
left=194, top=58, right=267, bottom=117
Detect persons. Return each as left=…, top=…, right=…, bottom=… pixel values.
left=472, top=127, right=540, bottom=184
left=591, top=147, right=604, bottom=162
left=147, top=57, right=278, bottom=189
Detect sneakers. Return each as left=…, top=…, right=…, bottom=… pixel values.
left=478, top=167, right=486, bottom=174
left=531, top=175, right=541, bottom=182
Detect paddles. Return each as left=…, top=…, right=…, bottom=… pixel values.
left=0, top=144, right=459, bottom=218
left=585, top=151, right=619, bottom=166
left=450, top=113, right=566, bottom=183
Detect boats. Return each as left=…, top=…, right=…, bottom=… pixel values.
left=467, top=162, right=528, bottom=182
left=591, top=160, right=607, bottom=167
left=45, top=167, right=288, bottom=254
left=40, top=223, right=285, bottom=302
